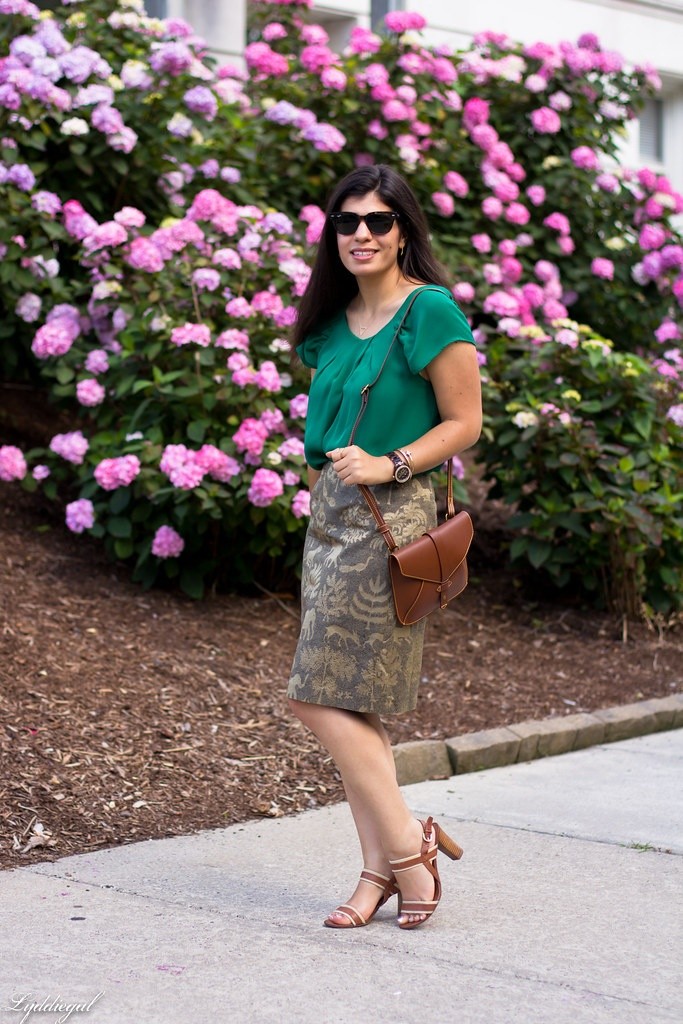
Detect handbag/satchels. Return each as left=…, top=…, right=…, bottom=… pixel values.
left=388, top=510, right=474, bottom=625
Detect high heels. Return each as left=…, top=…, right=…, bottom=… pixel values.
left=324, top=868, right=402, bottom=928
left=389, top=816, right=463, bottom=929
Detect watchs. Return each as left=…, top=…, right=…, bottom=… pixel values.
left=384, top=451, right=410, bottom=486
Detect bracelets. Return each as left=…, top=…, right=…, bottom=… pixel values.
left=394, top=445, right=415, bottom=474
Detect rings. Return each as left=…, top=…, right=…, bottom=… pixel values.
left=339, top=449, right=344, bottom=459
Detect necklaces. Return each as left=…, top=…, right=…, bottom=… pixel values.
left=355, top=279, right=401, bottom=335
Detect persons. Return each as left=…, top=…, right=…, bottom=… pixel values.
left=291, top=168, right=482, bottom=929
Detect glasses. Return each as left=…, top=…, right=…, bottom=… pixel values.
left=327, top=210, right=403, bottom=235
